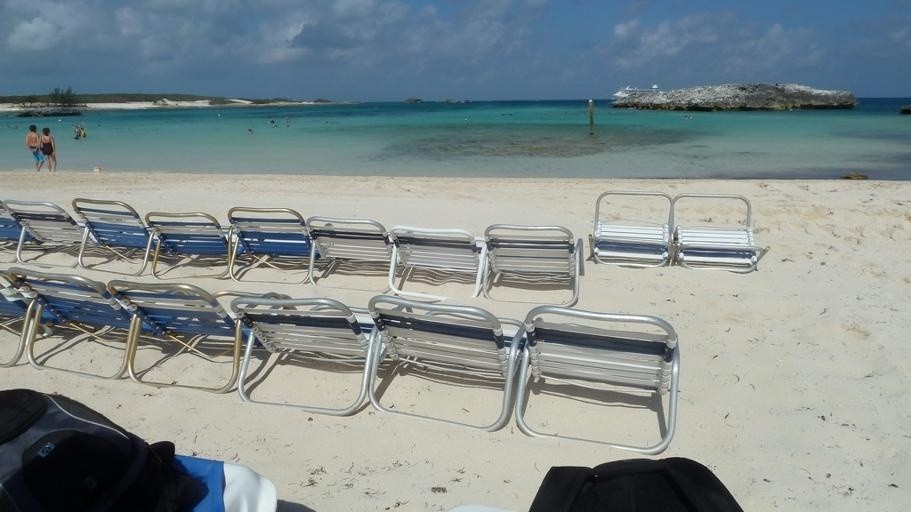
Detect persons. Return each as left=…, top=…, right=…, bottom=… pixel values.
left=39, top=127, right=57, bottom=172
left=248, top=129, right=253, bottom=135
left=71, top=124, right=86, bottom=140
left=25, top=125, right=45, bottom=171
left=270, top=120, right=277, bottom=128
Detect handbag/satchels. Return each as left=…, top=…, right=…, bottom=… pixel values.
left=0, top=390, right=210, bottom=511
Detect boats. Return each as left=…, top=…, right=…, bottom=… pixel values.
left=611, top=85, right=661, bottom=101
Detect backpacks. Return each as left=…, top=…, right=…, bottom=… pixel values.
left=529, top=458, right=742, bottom=512
